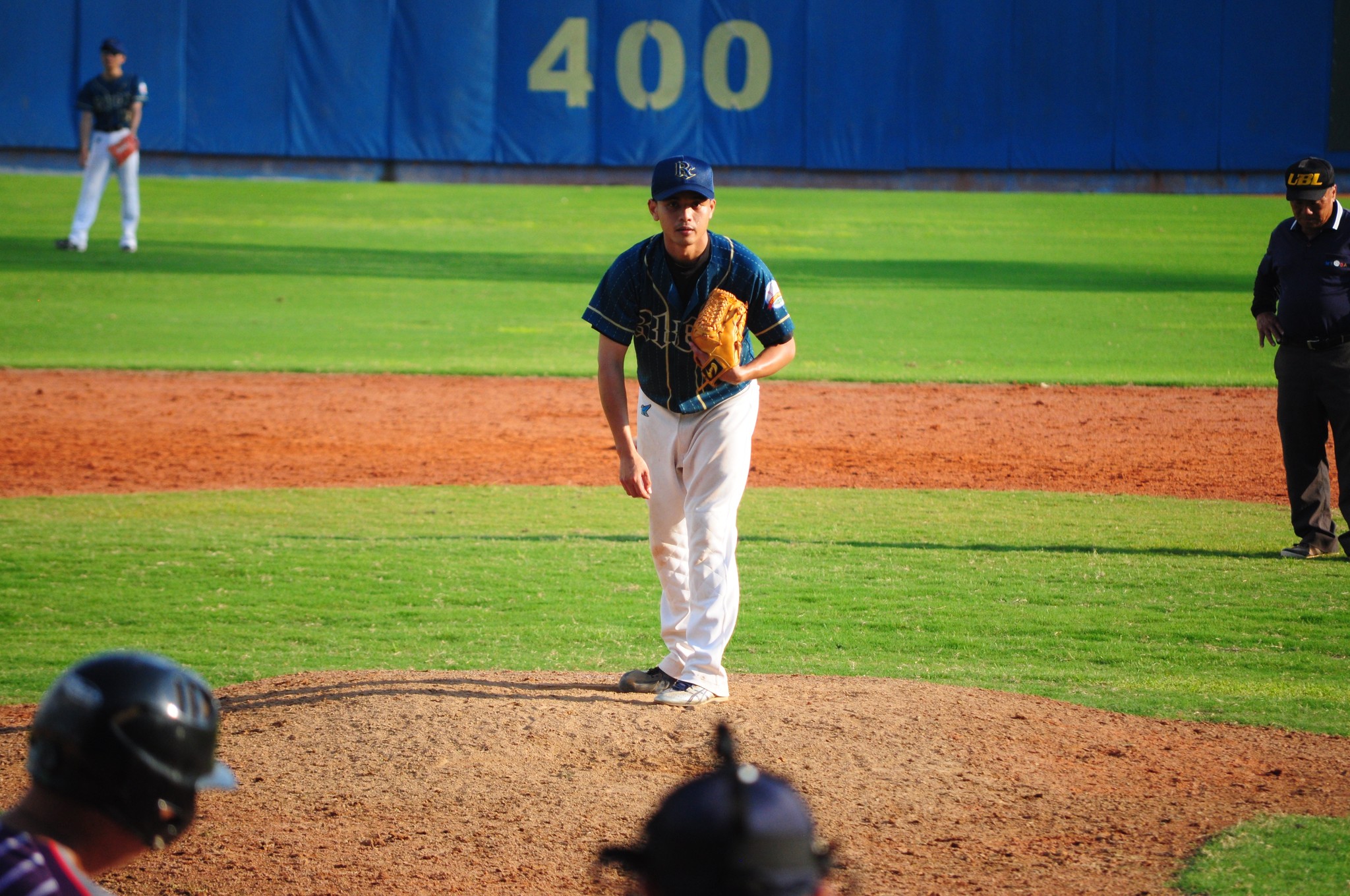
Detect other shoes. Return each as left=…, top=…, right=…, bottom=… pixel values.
left=122, top=247, right=135, bottom=253
left=55, top=239, right=84, bottom=254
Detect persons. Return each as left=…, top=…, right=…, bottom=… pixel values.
left=581, top=157, right=795, bottom=706
left=597, top=723, right=837, bottom=896
left=57, top=38, right=148, bottom=253
left=1251, top=156, right=1350, bottom=560
left=0, top=650, right=234, bottom=896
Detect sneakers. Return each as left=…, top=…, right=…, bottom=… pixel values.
left=1281, top=542, right=1340, bottom=558
left=618, top=665, right=677, bottom=692
left=656, top=680, right=727, bottom=705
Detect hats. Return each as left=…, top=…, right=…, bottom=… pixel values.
left=644, top=763, right=818, bottom=896
left=1284, top=158, right=1336, bottom=200
left=651, top=156, right=714, bottom=200
left=102, top=39, right=126, bottom=53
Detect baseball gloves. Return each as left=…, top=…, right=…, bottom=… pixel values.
left=690, top=288, right=748, bottom=386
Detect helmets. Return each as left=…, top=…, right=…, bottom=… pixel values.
left=28, top=651, right=236, bottom=845
